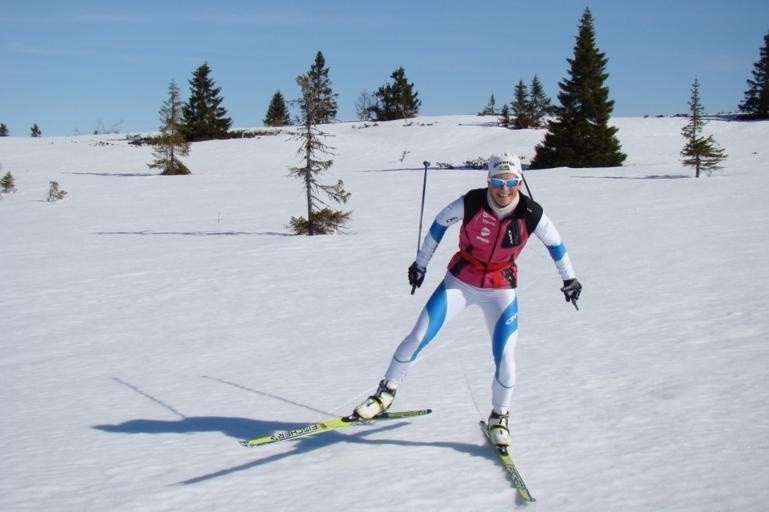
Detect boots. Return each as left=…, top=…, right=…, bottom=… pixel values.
left=487, top=411, right=510, bottom=448
left=353, top=380, right=398, bottom=421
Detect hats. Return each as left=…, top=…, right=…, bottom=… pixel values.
left=488, top=155, right=522, bottom=179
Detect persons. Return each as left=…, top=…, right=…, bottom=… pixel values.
left=352, top=154, right=583, bottom=455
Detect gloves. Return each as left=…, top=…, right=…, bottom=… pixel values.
left=561, top=278, right=581, bottom=302
left=407, top=261, right=425, bottom=288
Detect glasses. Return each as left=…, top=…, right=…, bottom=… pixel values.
left=488, top=177, right=521, bottom=189
